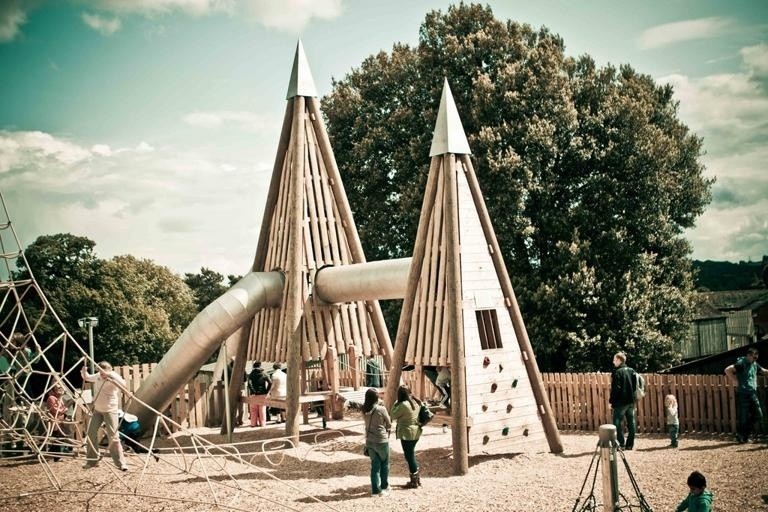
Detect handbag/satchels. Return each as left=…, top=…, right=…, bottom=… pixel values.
left=363, top=446, right=368, bottom=456
left=419, top=404, right=433, bottom=425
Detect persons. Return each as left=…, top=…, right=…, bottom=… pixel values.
left=676, top=471, right=713, bottom=512
left=724, top=349, right=768, bottom=441
left=46, top=378, right=75, bottom=462
left=362, top=384, right=423, bottom=495
left=0, top=333, right=41, bottom=448
left=435, top=363, right=451, bottom=409
left=80, top=362, right=159, bottom=471
left=364, top=351, right=381, bottom=387
left=607, top=352, right=646, bottom=451
left=221, top=355, right=287, bottom=428
left=663, top=394, right=680, bottom=449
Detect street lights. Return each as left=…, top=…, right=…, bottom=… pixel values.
left=77, top=317, right=99, bottom=375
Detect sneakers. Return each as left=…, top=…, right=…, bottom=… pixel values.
left=83, top=462, right=100, bottom=468
left=618, top=442, right=632, bottom=450
left=371, top=485, right=391, bottom=497
left=119, top=463, right=128, bottom=471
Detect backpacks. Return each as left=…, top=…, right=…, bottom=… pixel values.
left=634, top=373, right=646, bottom=402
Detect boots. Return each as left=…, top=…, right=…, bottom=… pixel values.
left=403, top=470, right=421, bottom=488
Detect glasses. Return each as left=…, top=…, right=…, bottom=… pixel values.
left=752, top=356, right=758, bottom=359
